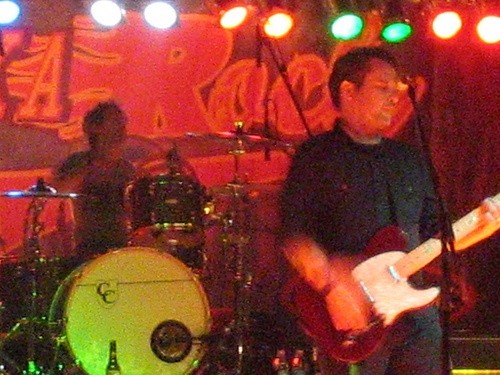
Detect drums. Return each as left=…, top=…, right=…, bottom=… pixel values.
left=49, top=246, right=213, bottom=375
left=0, top=251, right=86, bottom=323
left=125, top=173, right=206, bottom=250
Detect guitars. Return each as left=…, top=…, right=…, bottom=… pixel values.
left=282, top=190, right=500, bottom=365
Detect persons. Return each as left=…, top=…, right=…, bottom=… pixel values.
left=54, top=102, right=140, bottom=255
left=278, top=46, right=456, bottom=375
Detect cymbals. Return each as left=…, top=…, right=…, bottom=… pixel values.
left=209, top=184, right=278, bottom=200
left=1, top=190, right=85, bottom=200
left=184, top=132, right=297, bottom=153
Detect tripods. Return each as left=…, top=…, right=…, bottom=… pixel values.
left=202, top=122, right=275, bottom=374
left=1, top=202, right=71, bottom=374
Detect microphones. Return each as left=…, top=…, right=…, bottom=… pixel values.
left=254, top=22, right=265, bottom=69
